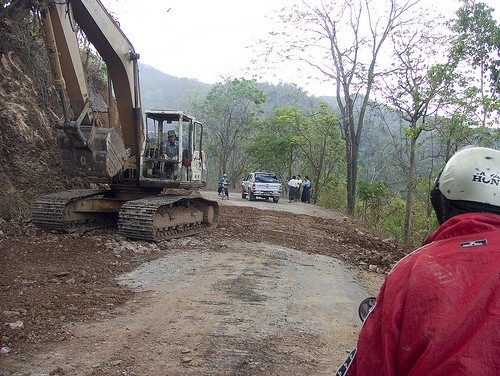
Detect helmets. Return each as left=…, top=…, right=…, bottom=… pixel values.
left=430, top=145, right=500, bottom=226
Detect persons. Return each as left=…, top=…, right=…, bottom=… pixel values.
left=301, top=176, right=311, bottom=204
left=287, top=176, right=298, bottom=202
left=296, top=175, right=303, bottom=201
left=160, top=130, right=182, bottom=178
left=217, top=174, right=230, bottom=200
left=333, top=148, right=500, bottom=376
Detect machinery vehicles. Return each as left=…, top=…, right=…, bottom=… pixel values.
left=30, top=0, right=220, bottom=242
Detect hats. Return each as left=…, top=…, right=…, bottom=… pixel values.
left=168, top=130, right=175, bottom=137
left=223, top=174, right=228, bottom=177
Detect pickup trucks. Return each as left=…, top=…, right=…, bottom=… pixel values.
left=241, top=172, right=283, bottom=204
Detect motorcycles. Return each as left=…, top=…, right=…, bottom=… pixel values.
left=218, top=182, right=231, bottom=201
